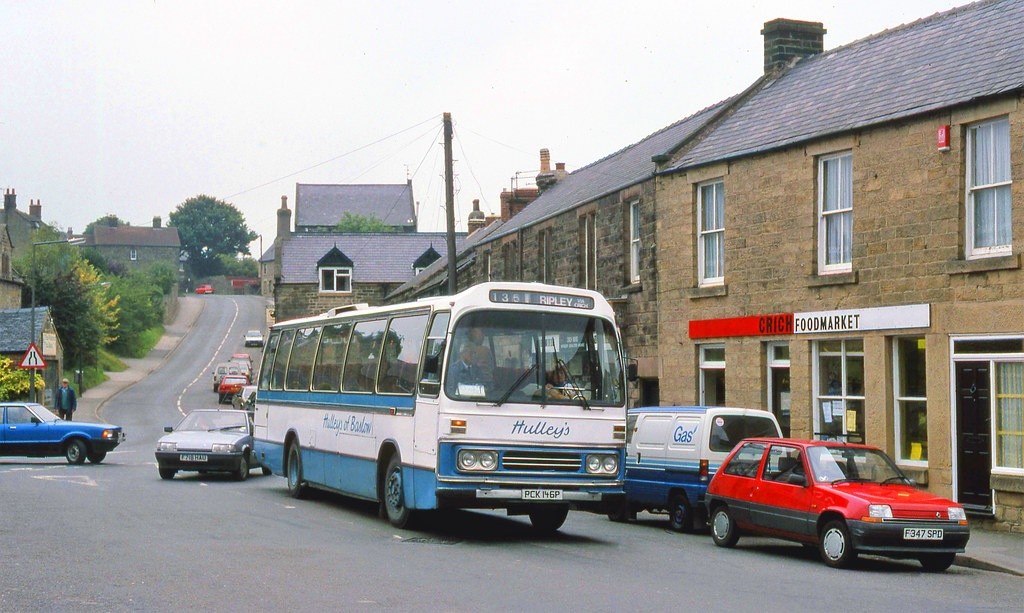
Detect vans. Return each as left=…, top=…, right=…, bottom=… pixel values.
left=607, top=405, right=784, bottom=533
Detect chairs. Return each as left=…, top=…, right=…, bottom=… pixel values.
left=773, top=458, right=805, bottom=484
left=826, top=462, right=850, bottom=482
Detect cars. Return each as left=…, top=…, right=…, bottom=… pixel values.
left=195, top=284, right=215, bottom=294
left=242, top=391, right=258, bottom=410
left=704, top=438, right=970, bottom=572
left=243, top=330, right=265, bottom=347
left=229, top=353, right=254, bottom=371
left=155, top=409, right=272, bottom=480
left=0, top=403, right=126, bottom=465
left=228, top=361, right=252, bottom=381
left=232, top=385, right=258, bottom=410
left=218, top=375, right=253, bottom=404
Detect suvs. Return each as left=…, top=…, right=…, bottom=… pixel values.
left=212, top=363, right=242, bottom=392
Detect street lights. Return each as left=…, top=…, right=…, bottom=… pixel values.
left=29, top=237, right=87, bottom=403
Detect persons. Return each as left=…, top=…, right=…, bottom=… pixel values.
left=54, top=378, right=77, bottom=421
left=445, top=342, right=490, bottom=400
left=545, top=369, right=589, bottom=401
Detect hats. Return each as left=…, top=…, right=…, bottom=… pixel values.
left=63, top=378, right=68, bottom=383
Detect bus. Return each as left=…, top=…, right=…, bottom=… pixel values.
left=253, top=282, right=640, bottom=533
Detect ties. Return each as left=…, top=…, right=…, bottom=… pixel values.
left=468, top=366, right=471, bottom=375
